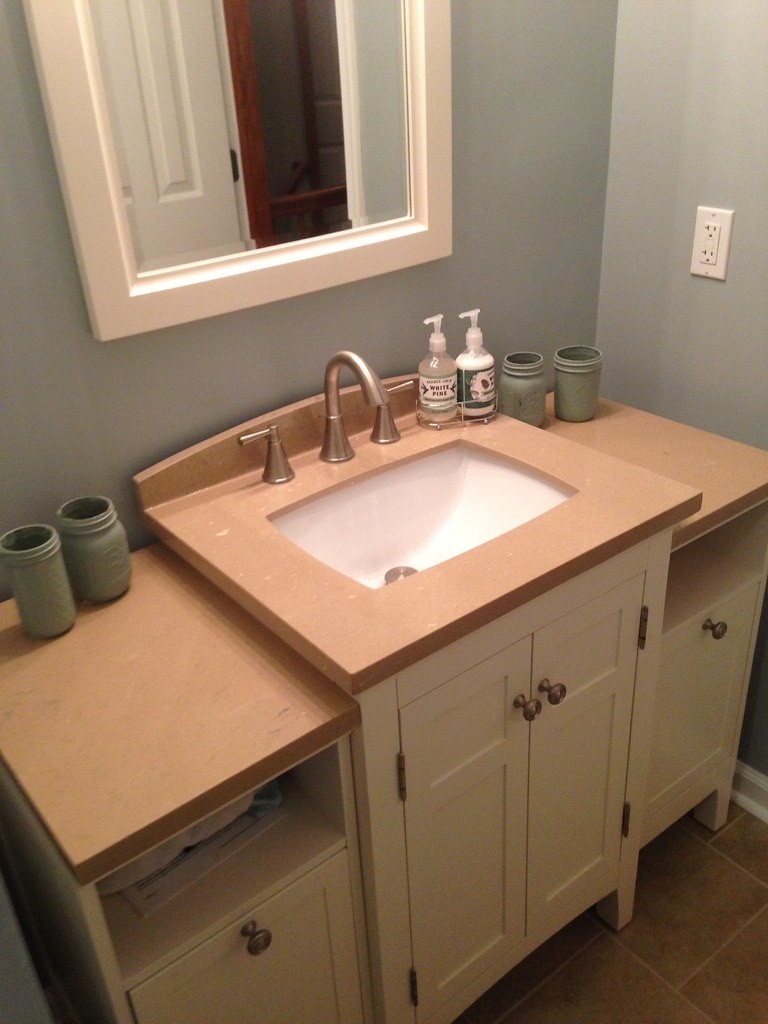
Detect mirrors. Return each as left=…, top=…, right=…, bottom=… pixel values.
left=23, top=0, right=452, bottom=343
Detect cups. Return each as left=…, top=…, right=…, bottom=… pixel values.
left=554, top=346, right=603, bottom=421
left=0, top=525, right=76, bottom=639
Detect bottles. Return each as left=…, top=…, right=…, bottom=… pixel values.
left=498, top=352, right=546, bottom=427
left=58, top=495, right=131, bottom=601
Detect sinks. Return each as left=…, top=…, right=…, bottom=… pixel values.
left=265, top=439, right=580, bottom=590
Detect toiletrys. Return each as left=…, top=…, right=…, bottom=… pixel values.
left=419, top=314, right=457, bottom=422
left=456, top=308, right=495, bottom=416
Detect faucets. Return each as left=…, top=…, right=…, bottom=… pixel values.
left=318, top=350, right=390, bottom=463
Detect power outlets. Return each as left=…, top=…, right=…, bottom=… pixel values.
left=691, top=206, right=736, bottom=282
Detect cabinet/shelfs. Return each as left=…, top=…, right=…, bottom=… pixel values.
left=0, top=499, right=768, bottom=1024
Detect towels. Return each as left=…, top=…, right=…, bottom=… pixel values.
left=95, top=784, right=254, bottom=898
